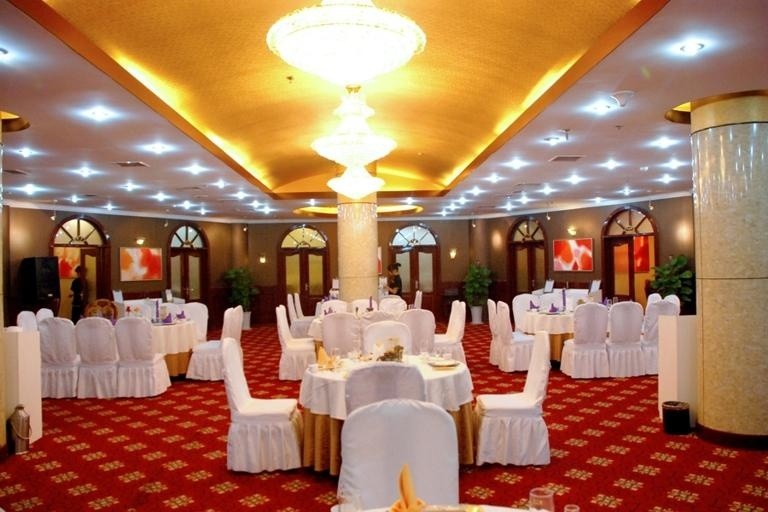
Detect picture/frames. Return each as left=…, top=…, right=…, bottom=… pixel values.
left=118, top=246, right=163, bottom=285
left=553, top=236, right=595, bottom=273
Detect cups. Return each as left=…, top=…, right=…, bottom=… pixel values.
left=608, top=300, right=612, bottom=308
left=613, top=297, right=618, bottom=303
left=444, top=348, right=452, bottom=359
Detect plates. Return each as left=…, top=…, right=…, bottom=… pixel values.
left=547, top=311, right=559, bottom=315
left=428, top=360, right=459, bottom=368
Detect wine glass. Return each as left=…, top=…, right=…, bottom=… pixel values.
left=329, top=348, right=341, bottom=373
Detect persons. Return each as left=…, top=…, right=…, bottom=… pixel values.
left=383, top=262, right=402, bottom=297
left=68, top=266, right=87, bottom=324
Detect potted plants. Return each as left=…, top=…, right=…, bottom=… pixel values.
left=222, top=265, right=260, bottom=332
left=462, top=260, right=494, bottom=325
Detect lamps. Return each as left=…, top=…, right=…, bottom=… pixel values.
left=311, top=119, right=397, bottom=166
left=334, top=88, right=373, bottom=121
left=266, top=0, right=427, bottom=94
left=326, top=160, right=385, bottom=201
